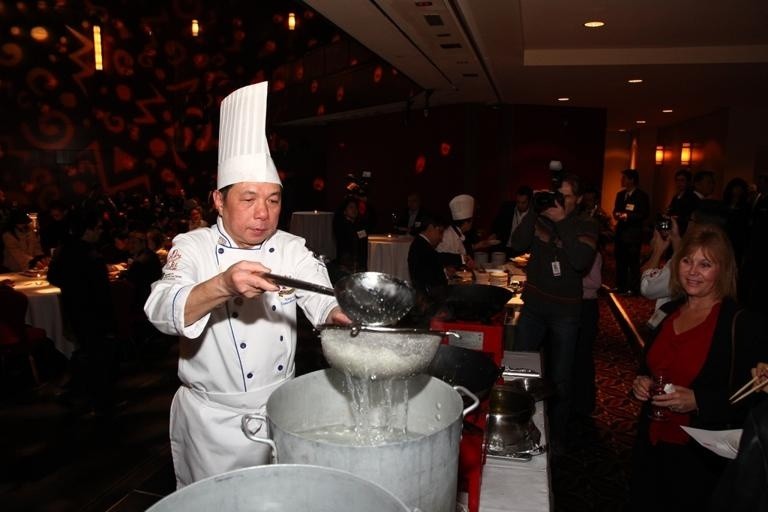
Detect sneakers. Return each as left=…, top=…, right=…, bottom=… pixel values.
left=607, top=288, right=637, bottom=297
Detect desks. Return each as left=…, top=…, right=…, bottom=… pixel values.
left=289, top=210, right=338, bottom=263
left=367, top=232, right=414, bottom=288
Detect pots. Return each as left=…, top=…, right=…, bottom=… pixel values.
left=241, top=367, right=480, bottom=512
left=145, top=463, right=422, bottom=512
left=429, top=345, right=504, bottom=406
left=428, top=283, right=515, bottom=322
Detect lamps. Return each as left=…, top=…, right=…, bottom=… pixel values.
left=681, top=142, right=690, bottom=164
left=655, top=144, right=664, bottom=165
left=191, top=19, right=199, bottom=37
left=289, top=13, right=295, bottom=30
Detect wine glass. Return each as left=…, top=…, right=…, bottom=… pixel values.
left=648, top=372, right=672, bottom=421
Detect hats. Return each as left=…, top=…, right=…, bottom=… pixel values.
left=217, top=82, right=283, bottom=191
left=449, top=195, right=474, bottom=220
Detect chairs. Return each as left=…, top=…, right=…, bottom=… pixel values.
left=1, top=352, right=43, bottom=387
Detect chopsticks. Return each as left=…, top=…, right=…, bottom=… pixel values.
left=728, top=367, right=768, bottom=404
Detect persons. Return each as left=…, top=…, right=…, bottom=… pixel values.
left=0, top=165, right=768, bottom=512
left=144, top=79, right=351, bottom=487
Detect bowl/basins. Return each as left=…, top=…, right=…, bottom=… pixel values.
left=491, top=252, right=506, bottom=267
left=475, top=252, right=487, bottom=268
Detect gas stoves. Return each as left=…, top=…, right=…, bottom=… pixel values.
left=429, top=307, right=504, bottom=367
left=460, top=407, right=488, bottom=512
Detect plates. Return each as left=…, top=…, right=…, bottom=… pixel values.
left=510, top=257, right=528, bottom=262
left=488, top=239, right=501, bottom=245
left=476, top=270, right=508, bottom=288
left=510, top=274, right=527, bottom=284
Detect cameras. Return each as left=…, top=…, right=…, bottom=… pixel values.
left=532, top=190, right=565, bottom=212
left=658, top=219, right=672, bottom=232
